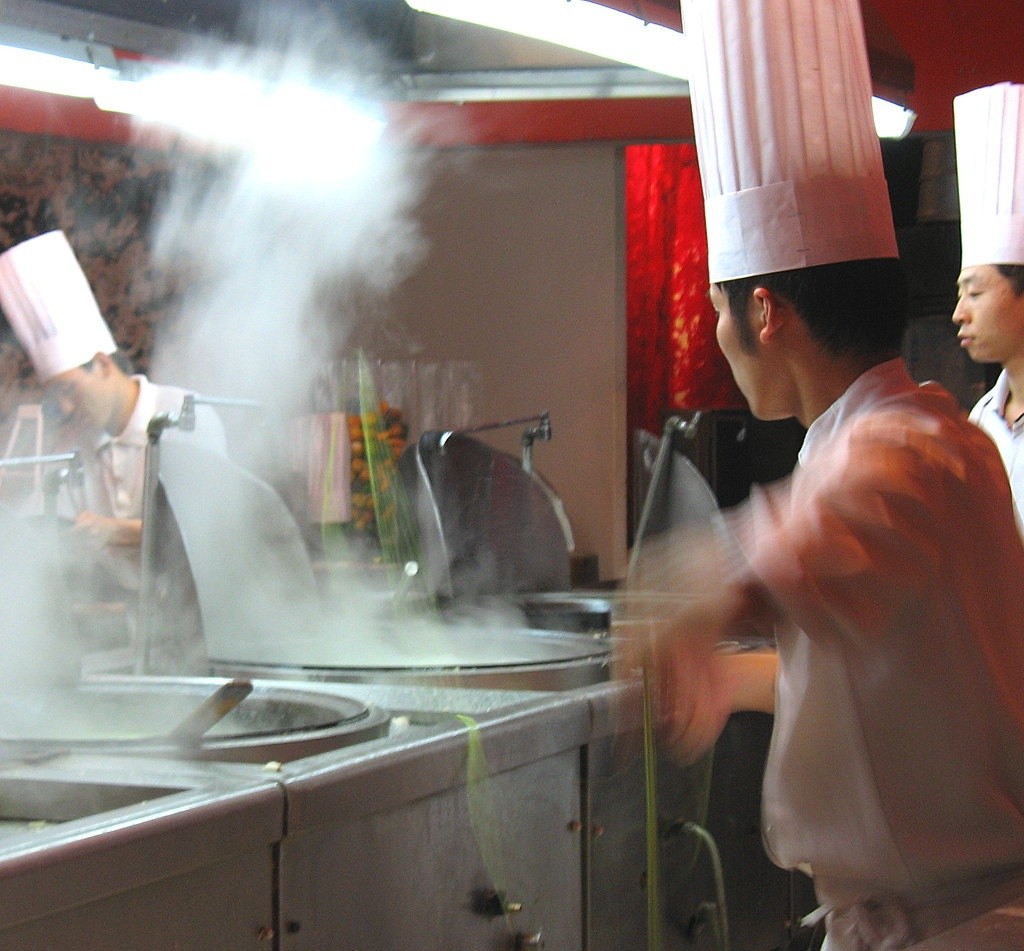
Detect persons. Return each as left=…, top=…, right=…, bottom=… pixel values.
left=952, top=211, right=1024, bottom=545
left=38, top=342, right=223, bottom=591
left=610, top=176, right=1024, bottom=950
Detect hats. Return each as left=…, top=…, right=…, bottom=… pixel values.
left=680, top=0, right=899, bottom=287
left=0, top=230, right=118, bottom=382
left=953, top=81, right=1024, bottom=270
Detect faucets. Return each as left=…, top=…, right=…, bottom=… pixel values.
left=522, top=411, right=551, bottom=447
left=147, top=396, right=195, bottom=444
left=664, top=410, right=704, bottom=440
left=42, top=451, right=84, bottom=494
